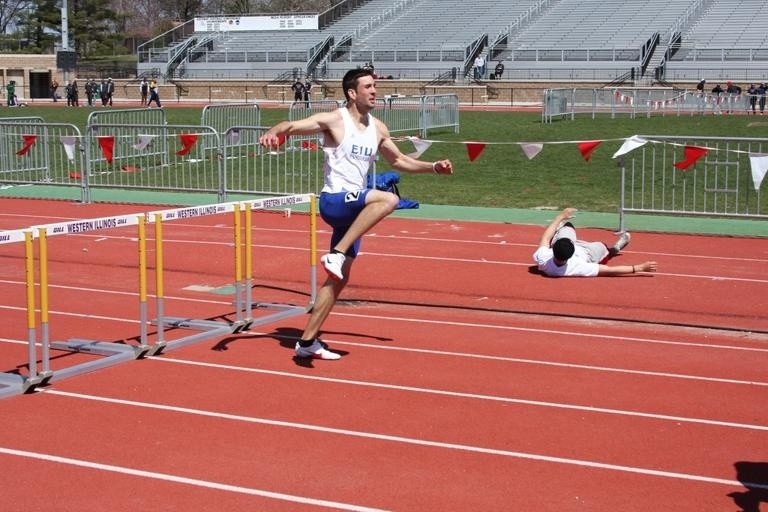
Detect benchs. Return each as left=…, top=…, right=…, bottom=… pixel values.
left=136, top=0, right=768, bottom=85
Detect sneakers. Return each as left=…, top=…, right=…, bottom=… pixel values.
left=614, top=232, right=630, bottom=252
left=294, top=339, right=341, bottom=360
left=320, top=252, right=346, bottom=282
left=67, top=104, right=113, bottom=107
left=712, top=110, right=765, bottom=115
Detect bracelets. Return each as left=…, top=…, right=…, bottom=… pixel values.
left=432, top=162, right=439, bottom=175
left=632, top=265, right=636, bottom=273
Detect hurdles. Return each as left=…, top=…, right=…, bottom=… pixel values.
left=0, top=192, right=315, bottom=400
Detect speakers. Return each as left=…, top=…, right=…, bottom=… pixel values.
left=57, top=51, right=76, bottom=68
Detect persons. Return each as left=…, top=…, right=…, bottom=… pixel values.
left=474, top=52, right=504, bottom=79
left=139, top=76, right=164, bottom=108
left=7, top=81, right=16, bottom=107
left=697, top=78, right=768, bottom=115
left=290, top=77, right=312, bottom=109
left=532, top=207, right=659, bottom=277
left=52, top=76, right=114, bottom=108
left=258, top=67, right=454, bottom=359
